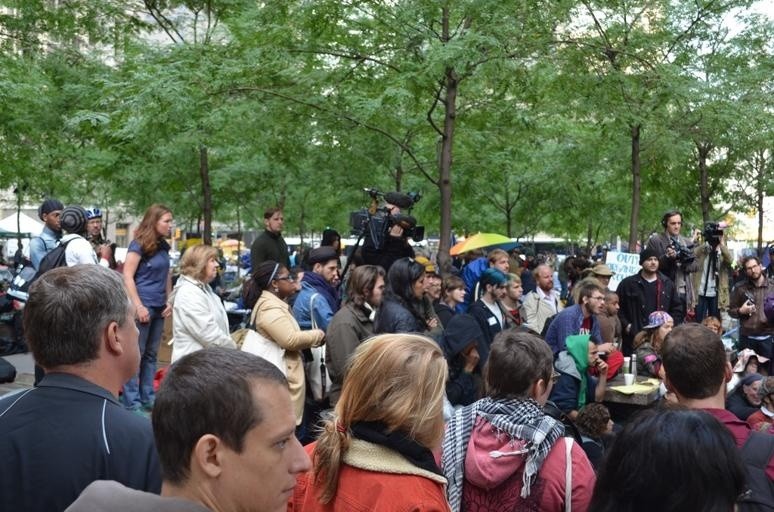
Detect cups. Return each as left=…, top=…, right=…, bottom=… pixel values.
left=624, top=373, right=635, bottom=385
left=622, top=357, right=631, bottom=376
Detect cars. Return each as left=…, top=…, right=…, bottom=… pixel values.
left=741, top=245, right=772, bottom=276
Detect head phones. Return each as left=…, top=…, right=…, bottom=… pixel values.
left=661, top=210, right=675, bottom=227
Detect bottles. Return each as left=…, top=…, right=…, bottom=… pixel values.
left=631, top=354, right=638, bottom=379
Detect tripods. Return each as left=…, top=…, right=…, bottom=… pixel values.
left=700, top=250, right=719, bottom=320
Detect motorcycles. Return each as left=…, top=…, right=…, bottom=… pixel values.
left=0, top=265, right=25, bottom=357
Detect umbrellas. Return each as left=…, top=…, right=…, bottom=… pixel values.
left=450, top=230, right=513, bottom=257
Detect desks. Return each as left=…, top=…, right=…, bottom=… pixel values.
left=603, top=349, right=737, bottom=420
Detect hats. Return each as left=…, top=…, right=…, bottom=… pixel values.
left=57, top=205, right=88, bottom=234
left=641, top=310, right=674, bottom=331
left=591, top=265, right=616, bottom=276
left=639, top=249, right=660, bottom=266
left=730, top=348, right=771, bottom=373
left=414, top=256, right=435, bottom=273
left=39, top=199, right=64, bottom=221
left=308, top=245, right=339, bottom=265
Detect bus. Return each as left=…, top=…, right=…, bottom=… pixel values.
left=170, top=226, right=255, bottom=255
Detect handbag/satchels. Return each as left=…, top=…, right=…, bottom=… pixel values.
left=304, top=340, right=334, bottom=406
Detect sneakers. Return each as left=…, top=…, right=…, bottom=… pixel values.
left=127, top=407, right=152, bottom=418
left=142, top=401, right=154, bottom=410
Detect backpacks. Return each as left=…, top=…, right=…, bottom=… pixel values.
left=735, top=429, right=774, bottom=512
left=37, top=237, right=80, bottom=277
left=240, top=298, right=288, bottom=383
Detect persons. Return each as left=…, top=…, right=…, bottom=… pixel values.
left=694, top=234, right=734, bottom=321
left=320, top=229, right=343, bottom=268
left=30, top=198, right=64, bottom=271
left=644, top=209, right=697, bottom=326
left=1, top=245, right=774, bottom=511
left=86, top=208, right=117, bottom=268
left=362, top=202, right=415, bottom=268
left=250, top=207, right=291, bottom=273
left=56, top=206, right=113, bottom=268
left=122, top=203, right=173, bottom=415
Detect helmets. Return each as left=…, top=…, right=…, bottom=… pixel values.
left=84, top=208, right=102, bottom=220
